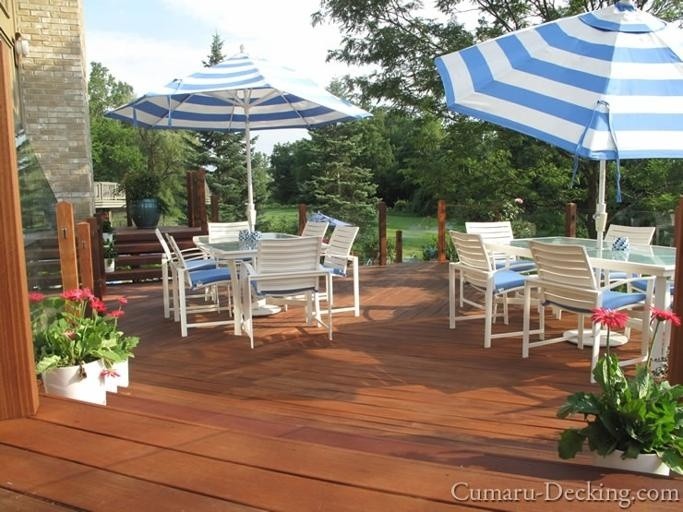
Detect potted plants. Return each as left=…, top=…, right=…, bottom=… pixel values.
left=102, top=238, right=118, bottom=273
left=112, top=165, right=172, bottom=229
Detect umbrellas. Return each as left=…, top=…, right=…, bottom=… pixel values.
left=431, top=1, right=683, bottom=348
left=103, top=42, right=375, bottom=317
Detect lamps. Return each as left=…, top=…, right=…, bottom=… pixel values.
left=13, top=31, right=30, bottom=68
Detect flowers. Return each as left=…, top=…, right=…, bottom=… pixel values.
left=101, top=210, right=111, bottom=232
left=28, top=287, right=140, bottom=378
left=556, top=308, right=682, bottom=476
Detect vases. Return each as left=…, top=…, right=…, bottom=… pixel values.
left=41, top=353, right=130, bottom=407
left=603, top=446, right=671, bottom=477
left=102, top=233, right=113, bottom=241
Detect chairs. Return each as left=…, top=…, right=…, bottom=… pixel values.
left=447, top=222, right=676, bottom=383
left=154, top=212, right=360, bottom=349
